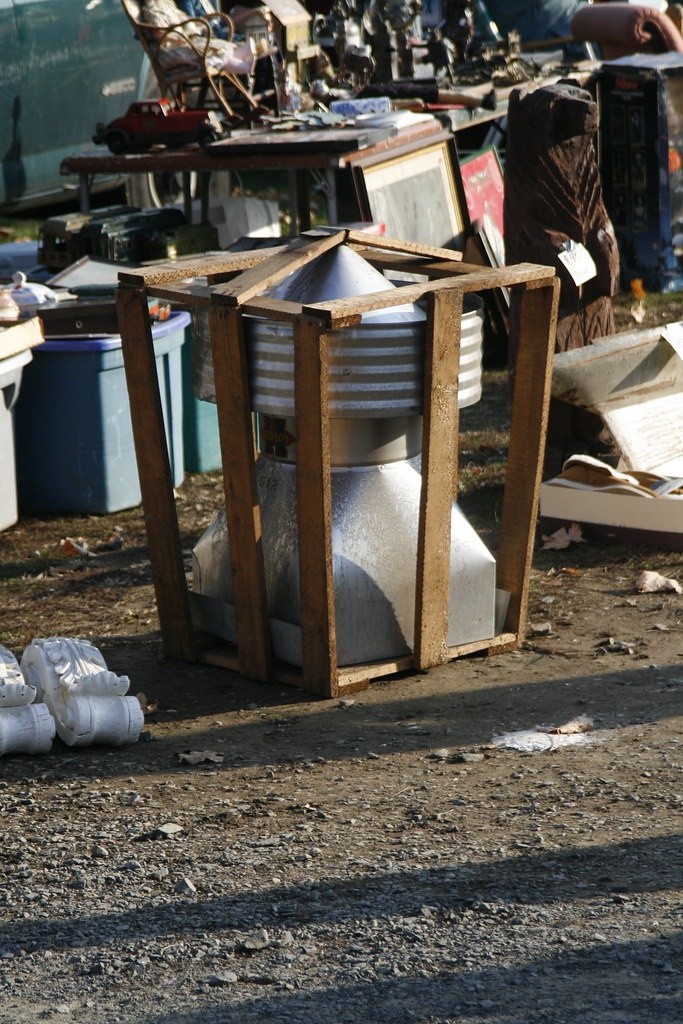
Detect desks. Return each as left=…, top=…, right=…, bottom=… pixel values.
left=59, top=69, right=592, bottom=226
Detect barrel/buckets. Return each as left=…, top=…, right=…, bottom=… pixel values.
left=10, top=311, right=191, bottom=515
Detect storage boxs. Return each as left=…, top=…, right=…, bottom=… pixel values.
left=13, top=310, right=191, bottom=515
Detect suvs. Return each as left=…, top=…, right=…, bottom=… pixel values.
left=93, top=97, right=220, bottom=155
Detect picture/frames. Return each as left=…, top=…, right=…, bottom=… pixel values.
left=462, top=146, right=509, bottom=306
left=350, top=127, right=474, bottom=254
